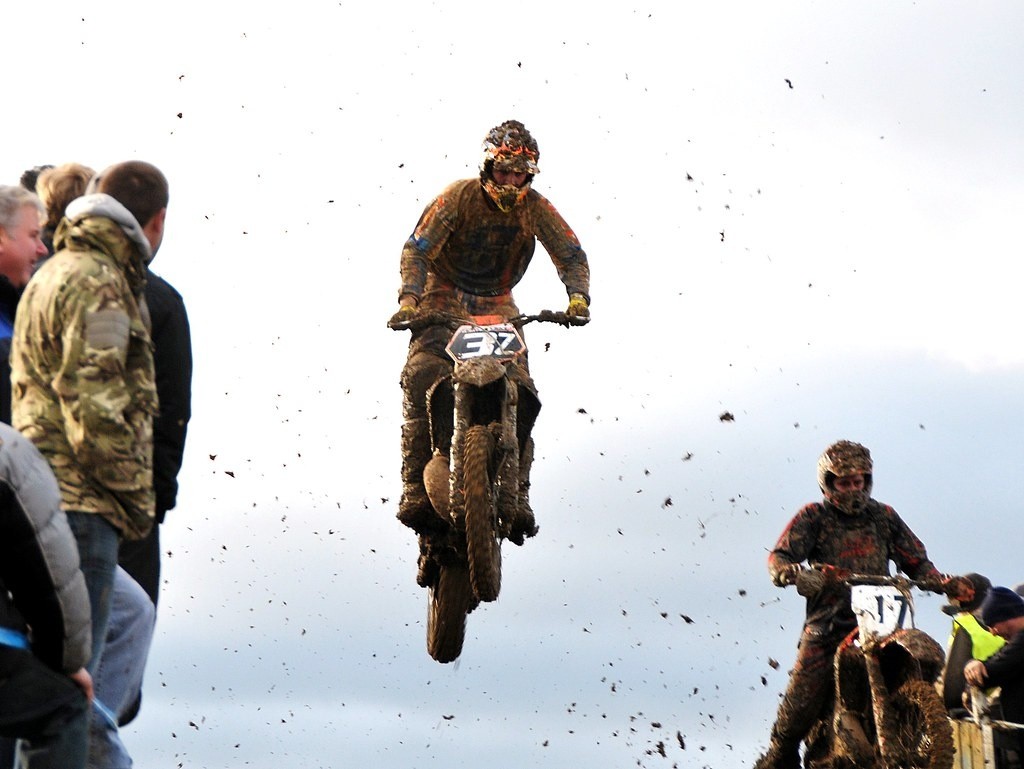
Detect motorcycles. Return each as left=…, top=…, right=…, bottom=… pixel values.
left=387, top=309, right=590, bottom=663
left=780, top=565, right=954, bottom=768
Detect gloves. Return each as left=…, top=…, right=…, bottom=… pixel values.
left=390, top=306, right=420, bottom=331
left=566, top=296, right=590, bottom=326
left=797, top=569, right=827, bottom=598
left=942, top=576, right=975, bottom=601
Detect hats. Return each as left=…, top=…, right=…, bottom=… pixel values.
left=942, top=573, right=990, bottom=615
left=982, top=586, right=1024, bottom=625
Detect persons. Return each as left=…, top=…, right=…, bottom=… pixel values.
left=756, top=440, right=975, bottom=769
left=941, top=571, right=1024, bottom=769
left=0, top=158, right=192, bottom=769
left=389, top=119, right=590, bottom=546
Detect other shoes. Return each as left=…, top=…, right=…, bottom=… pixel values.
left=399, top=499, right=435, bottom=533
left=516, top=495, right=535, bottom=533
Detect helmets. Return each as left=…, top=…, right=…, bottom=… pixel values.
left=817, top=440, right=873, bottom=513
left=479, top=120, right=540, bottom=213
